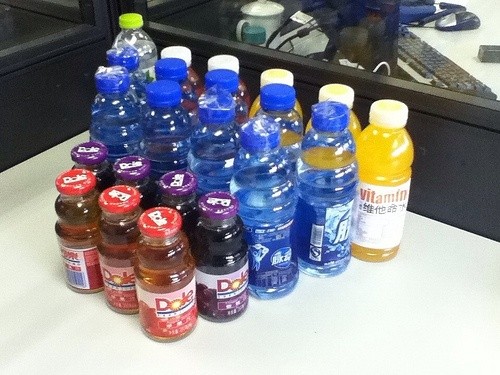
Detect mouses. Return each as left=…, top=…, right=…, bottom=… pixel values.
left=434, top=10, right=481, bottom=29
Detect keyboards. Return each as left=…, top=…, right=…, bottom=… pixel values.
left=393, top=24, right=498, bottom=100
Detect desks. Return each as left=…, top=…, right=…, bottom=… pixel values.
left=0, top=130, right=500, bottom=375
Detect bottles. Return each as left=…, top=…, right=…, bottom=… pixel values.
left=205, top=53, right=250, bottom=127
left=54, top=169, right=103, bottom=295
left=243, top=6, right=387, bottom=76
left=139, top=81, right=190, bottom=181
left=305, top=84, right=362, bottom=164
left=154, top=44, right=203, bottom=113
left=299, top=102, right=359, bottom=279
left=253, top=84, right=303, bottom=181
left=217, top=0, right=247, bottom=41
left=155, top=170, right=197, bottom=257
left=89, top=66, right=145, bottom=186
left=228, top=118, right=299, bottom=300
left=133, top=206, right=198, bottom=343
left=350, top=98, right=414, bottom=263
left=69, top=139, right=161, bottom=213
left=249, top=68, right=304, bottom=135
left=99, top=185, right=144, bottom=314
left=188, top=95, right=240, bottom=198
left=192, top=191, right=249, bottom=323
left=105, top=13, right=158, bottom=106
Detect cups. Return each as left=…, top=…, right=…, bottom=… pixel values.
left=236, top=0, right=285, bottom=47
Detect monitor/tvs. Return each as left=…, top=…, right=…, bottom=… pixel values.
left=301, top=0, right=401, bottom=76
